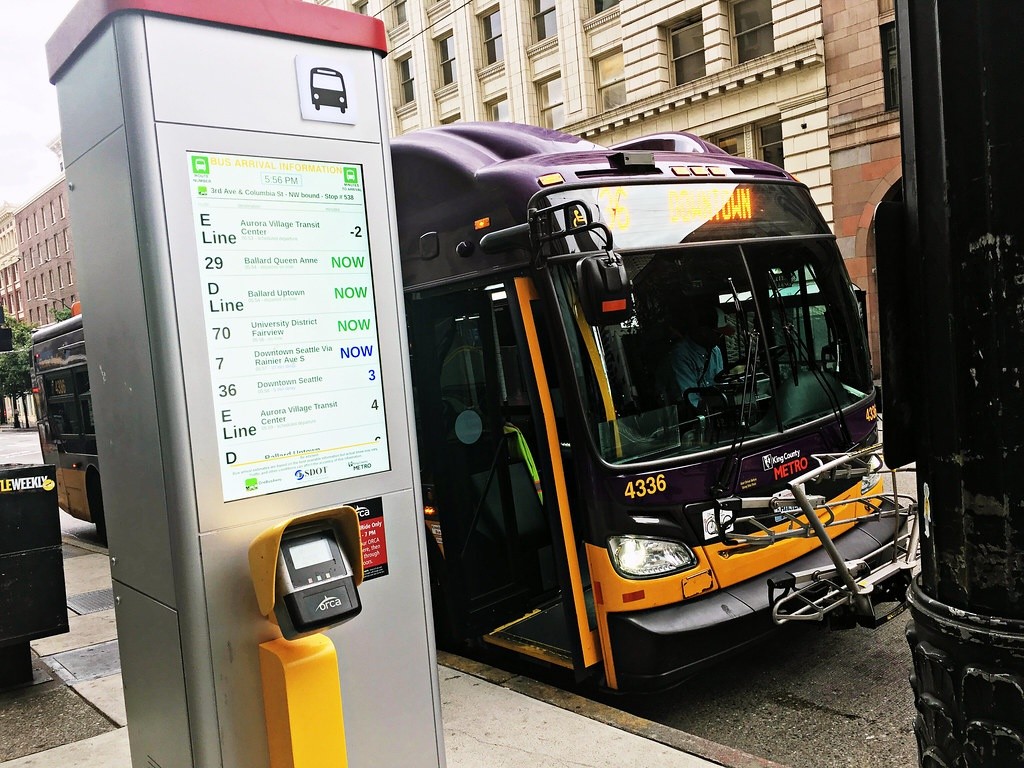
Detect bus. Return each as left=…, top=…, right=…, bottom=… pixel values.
left=27, top=120, right=918, bottom=712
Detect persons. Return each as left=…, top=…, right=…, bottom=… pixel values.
left=667, top=303, right=725, bottom=409
